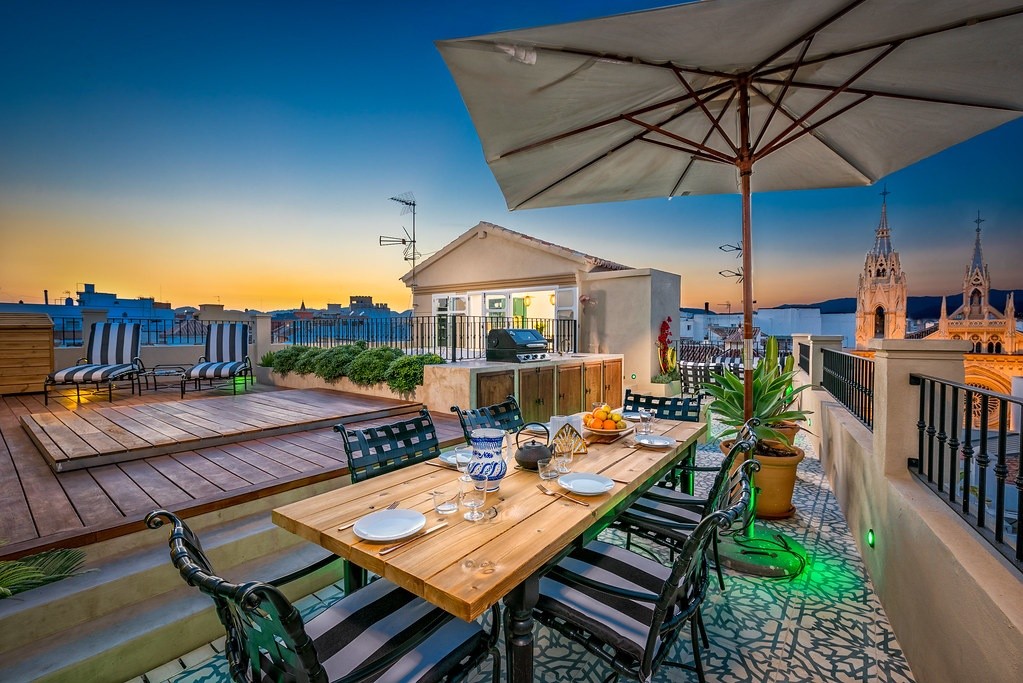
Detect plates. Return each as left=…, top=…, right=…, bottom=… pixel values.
left=525, top=423, right=552, bottom=434
left=621, top=412, right=651, bottom=422
left=635, top=434, right=677, bottom=448
left=352, top=508, right=426, bottom=541
left=555, top=472, right=615, bottom=496
left=439, top=449, right=472, bottom=468
left=582, top=419, right=635, bottom=435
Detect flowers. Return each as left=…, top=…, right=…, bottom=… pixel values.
left=652, top=316, right=680, bottom=383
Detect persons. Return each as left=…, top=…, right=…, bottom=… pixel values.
left=658, top=320, right=673, bottom=374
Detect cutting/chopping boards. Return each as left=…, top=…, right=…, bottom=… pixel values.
left=581, top=420, right=643, bottom=447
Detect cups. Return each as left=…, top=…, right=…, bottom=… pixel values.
left=550, top=415, right=582, bottom=443
left=634, top=423, right=648, bottom=437
left=433, top=487, right=461, bottom=514
left=537, top=459, right=560, bottom=480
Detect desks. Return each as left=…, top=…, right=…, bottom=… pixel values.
left=138, top=364, right=197, bottom=396
left=271, top=414, right=710, bottom=683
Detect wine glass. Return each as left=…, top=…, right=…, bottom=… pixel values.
left=553, top=438, right=574, bottom=474
left=455, top=446, right=488, bottom=521
left=593, top=401, right=655, bottom=435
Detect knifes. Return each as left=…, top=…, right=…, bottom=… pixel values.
left=520, top=432, right=547, bottom=439
left=425, top=460, right=457, bottom=471
left=378, top=522, right=449, bottom=555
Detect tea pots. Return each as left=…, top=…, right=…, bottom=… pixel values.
left=514, top=422, right=557, bottom=471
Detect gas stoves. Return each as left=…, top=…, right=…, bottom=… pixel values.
left=486, top=329, right=552, bottom=363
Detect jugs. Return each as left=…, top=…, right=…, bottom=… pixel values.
left=468, top=428, right=513, bottom=493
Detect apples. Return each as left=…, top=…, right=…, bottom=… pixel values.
left=583, top=407, right=617, bottom=429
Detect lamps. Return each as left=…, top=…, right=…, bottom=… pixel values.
left=550, top=294, right=556, bottom=305
left=524, top=295, right=531, bottom=307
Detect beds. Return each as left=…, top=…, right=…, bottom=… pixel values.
left=181, top=324, right=255, bottom=399
left=44, top=322, right=149, bottom=408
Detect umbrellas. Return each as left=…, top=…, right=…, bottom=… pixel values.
left=429, top=0, right=1020, bottom=535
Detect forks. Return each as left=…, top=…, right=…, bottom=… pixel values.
left=537, top=483, right=588, bottom=506
left=339, top=500, right=400, bottom=531
left=620, top=439, right=667, bottom=453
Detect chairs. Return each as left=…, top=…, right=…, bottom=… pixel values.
left=448, top=397, right=527, bottom=445
left=594, top=416, right=761, bottom=590
left=532, top=459, right=760, bottom=683
left=613, top=388, right=698, bottom=563
left=145, top=511, right=502, bottom=683
left=332, top=406, right=440, bottom=594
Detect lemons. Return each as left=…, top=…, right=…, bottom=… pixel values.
left=601, top=405, right=626, bottom=429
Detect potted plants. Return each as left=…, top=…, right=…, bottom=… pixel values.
left=700, top=330, right=817, bottom=519
